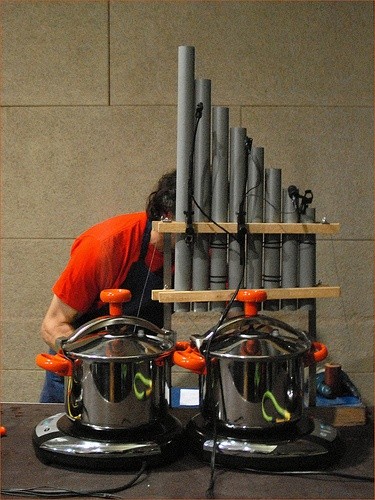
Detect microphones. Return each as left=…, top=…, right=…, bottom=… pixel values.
left=287, top=184, right=302, bottom=216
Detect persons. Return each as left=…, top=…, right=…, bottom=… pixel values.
left=38, top=170, right=176, bottom=402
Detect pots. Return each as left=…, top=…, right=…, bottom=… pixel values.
left=35, top=288, right=192, bottom=434
left=173, top=289, right=328, bottom=440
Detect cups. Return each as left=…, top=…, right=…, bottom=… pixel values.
left=325, top=362, right=342, bottom=388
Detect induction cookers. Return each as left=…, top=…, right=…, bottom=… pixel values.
left=187, top=411, right=341, bottom=473
left=32, top=409, right=184, bottom=468
